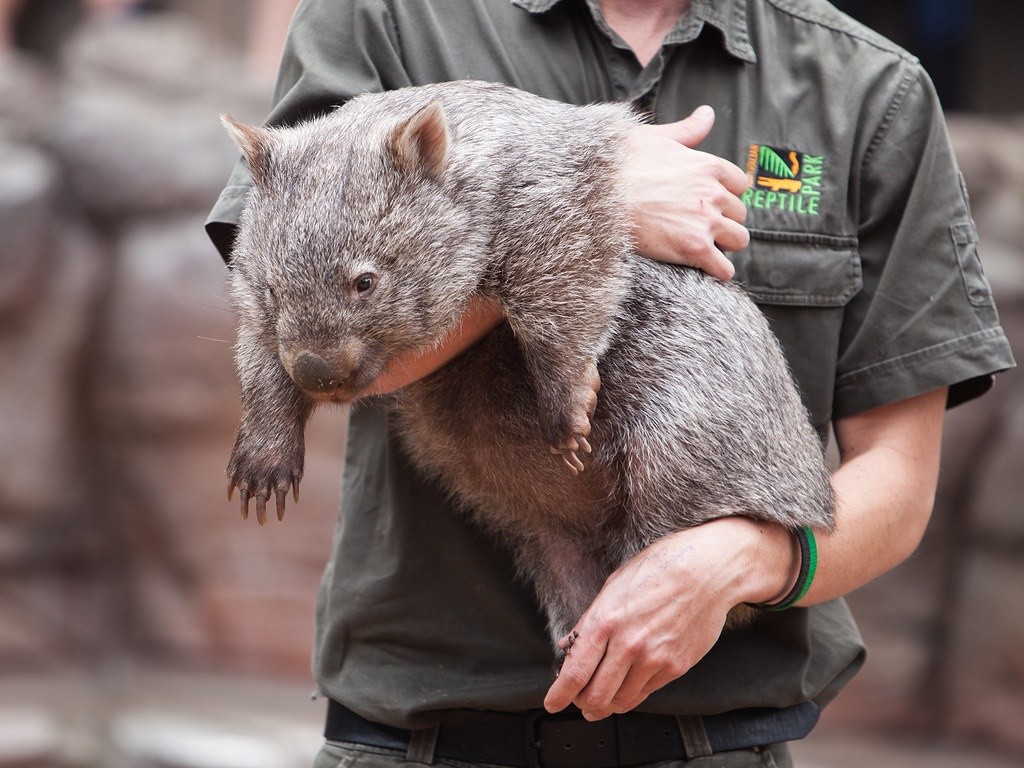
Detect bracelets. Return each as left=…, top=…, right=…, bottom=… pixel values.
left=743, top=525, right=819, bottom=612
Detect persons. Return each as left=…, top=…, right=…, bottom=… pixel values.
left=205, top=0, right=1018, bottom=768
